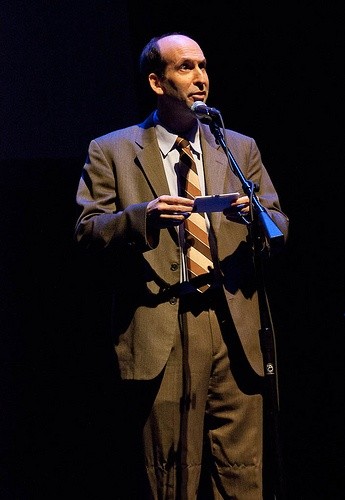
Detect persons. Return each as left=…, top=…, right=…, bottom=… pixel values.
left=74, top=34, right=290, bottom=499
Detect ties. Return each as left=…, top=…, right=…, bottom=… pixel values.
left=173, top=136, right=217, bottom=294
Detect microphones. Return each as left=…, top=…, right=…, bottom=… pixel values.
left=190, top=101, right=220, bottom=119
